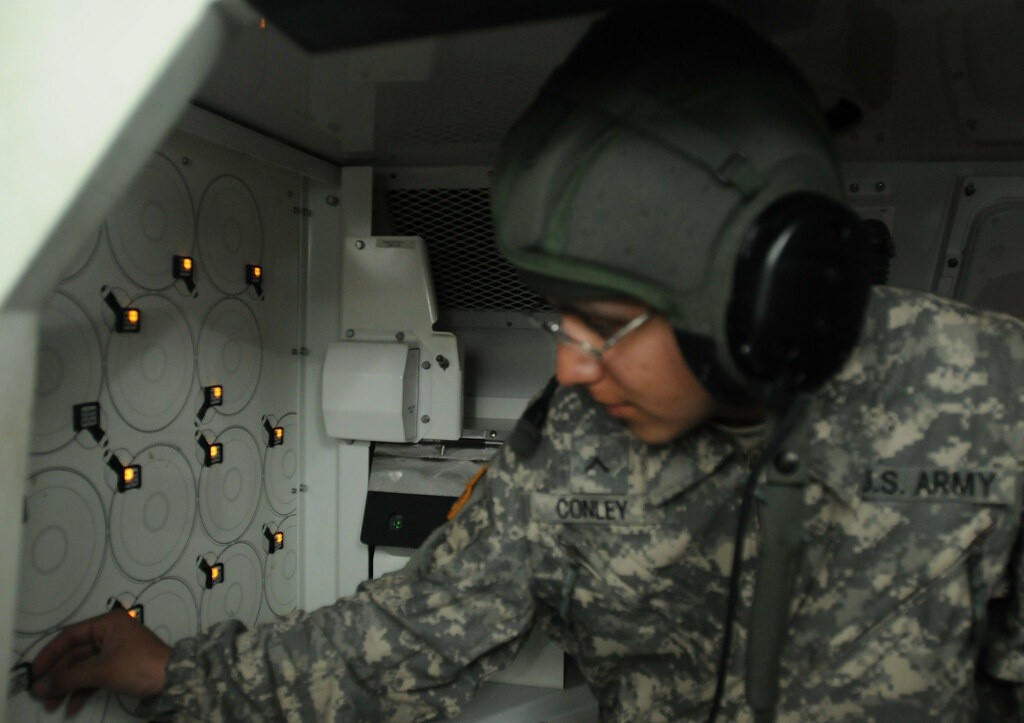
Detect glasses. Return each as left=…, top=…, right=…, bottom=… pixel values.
left=527, top=299, right=657, bottom=355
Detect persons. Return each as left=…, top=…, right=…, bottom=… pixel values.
left=26, top=0, right=1024, bottom=723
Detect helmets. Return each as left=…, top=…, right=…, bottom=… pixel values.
left=493, top=6, right=848, bottom=414
